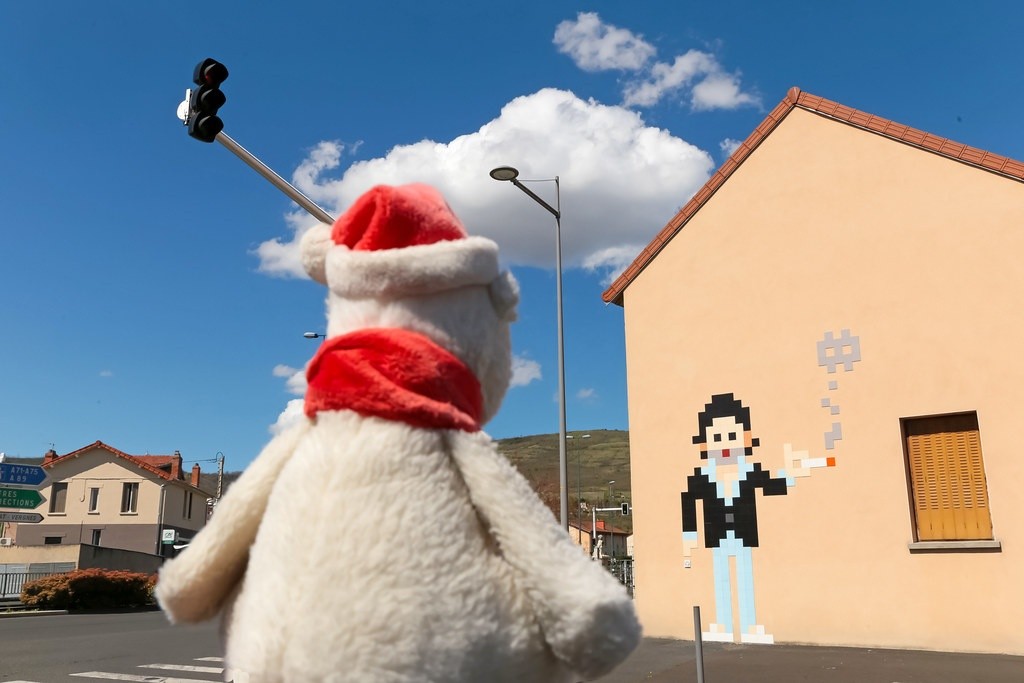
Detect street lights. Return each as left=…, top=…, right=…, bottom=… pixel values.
left=489, top=167, right=572, bottom=534
left=565, top=435, right=592, bottom=544
left=608, top=480, right=615, bottom=560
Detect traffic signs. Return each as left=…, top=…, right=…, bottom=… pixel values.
left=0, top=488, right=48, bottom=510
left=0, top=463, right=53, bottom=487
left=0, top=512, right=45, bottom=523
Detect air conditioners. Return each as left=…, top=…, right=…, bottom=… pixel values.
left=0, top=538, right=11, bottom=546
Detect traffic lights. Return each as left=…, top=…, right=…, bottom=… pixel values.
left=622, top=502, right=629, bottom=515
left=187, top=56, right=228, bottom=143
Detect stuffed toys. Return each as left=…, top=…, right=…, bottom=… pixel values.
left=149, top=181, right=644, bottom=683
